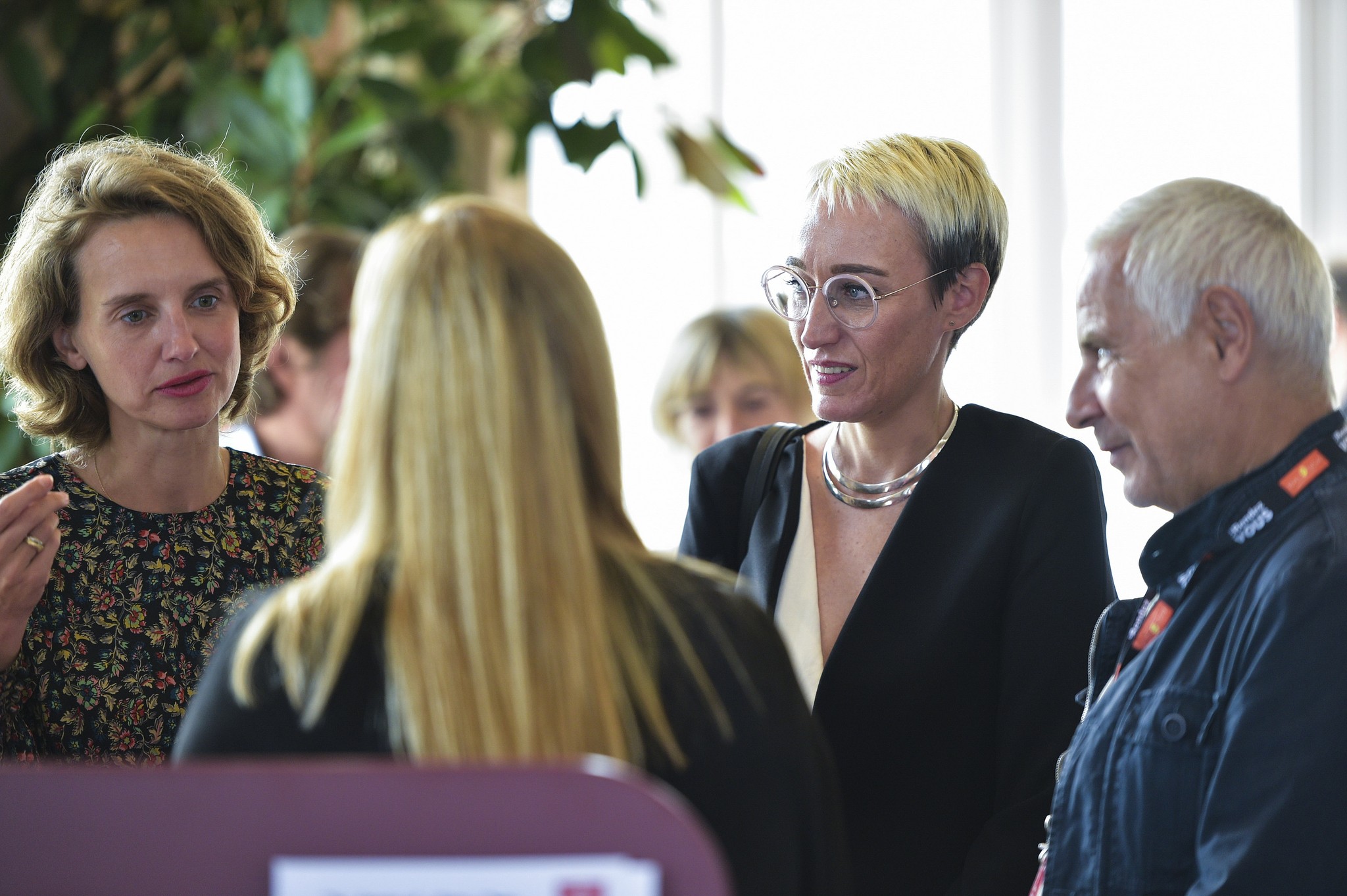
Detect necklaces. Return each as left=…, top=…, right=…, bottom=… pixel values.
left=818, top=401, right=960, bottom=511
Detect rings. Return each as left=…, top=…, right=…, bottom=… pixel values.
left=26, top=535, right=44, bottom=553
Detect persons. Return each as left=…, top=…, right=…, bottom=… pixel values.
left=1042, top=176, right=1347, bottom=894
left=653, top=302, right=815, bottom=460
left=0, top=133, right=332, bottom=763
left=176, top=192, right=848, bottom=894
left=672, top=132, right=1117, bottom=895
left=210, top=220, right=377, bottom=469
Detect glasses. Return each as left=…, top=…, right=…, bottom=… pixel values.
left=760, top=265, right=961, bottom=331
